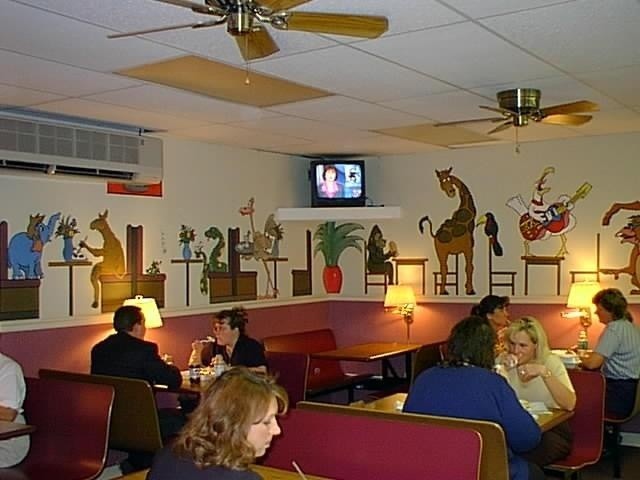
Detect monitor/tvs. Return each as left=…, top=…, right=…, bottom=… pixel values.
left=311, top=160, right=365, bottom=207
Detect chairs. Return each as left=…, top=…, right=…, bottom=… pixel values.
left=365, top=234, right=600, bottom=296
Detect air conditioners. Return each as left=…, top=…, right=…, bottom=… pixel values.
left=0, top=115, right=164, bottom=185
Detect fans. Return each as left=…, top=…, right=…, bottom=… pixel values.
left=432, top=89, right=600, bottom=135
left=107, top=0, right=390, bottom=62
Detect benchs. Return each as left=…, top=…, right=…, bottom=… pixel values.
left=295, top=401, right=510, bottom=480
left=252, top=409, right=482, bottom=477
left=263, top=328, right=372, bottom=401
left=265, top=352, right=311, bottom=412
left=1, top=377, right=115, bottom=479
left=601, top=389, right=639, bottom=421
left=544, top=369, right=610, bottom=471
left=42, top=367, right=167, bottom=454
left=404, top=341, right=445, bottom=389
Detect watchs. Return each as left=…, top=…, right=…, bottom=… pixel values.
left=541, top=369, right=553, bottom=379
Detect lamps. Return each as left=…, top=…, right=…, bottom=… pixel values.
left=384, top=284, right=415, bottom=321
left=560, top=282, right=600, bottom=327
left=122, top=295, right=163, bottom=328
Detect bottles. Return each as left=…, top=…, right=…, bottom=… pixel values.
left=189, top=354, right=224, bottom=384
left=578, top=329, right=588, bottom=349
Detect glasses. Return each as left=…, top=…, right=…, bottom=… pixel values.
left=213, top=326, right=232, bottom=335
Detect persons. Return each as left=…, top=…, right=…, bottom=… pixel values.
left=143, top=367, right=290, bottom=480
left=493, top=315, right=577, bottom=467
left=319, top=165, right=346, bottom=198
left=0, top=353, right=30, bottom=468
left=187, top=310, right=266, bottom=370
left=89, top=304, right=187, bottom=476
left=469, top=294, right=511, bottom=333
left=401, top=316, right=543, bottom=480
left=568, top=288, right=639, bottom=418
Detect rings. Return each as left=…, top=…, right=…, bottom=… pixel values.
left=521, top=371, right=525, bottom=375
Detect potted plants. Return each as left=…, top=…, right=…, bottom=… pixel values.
left=313, top=221, right=365, bottom=295
